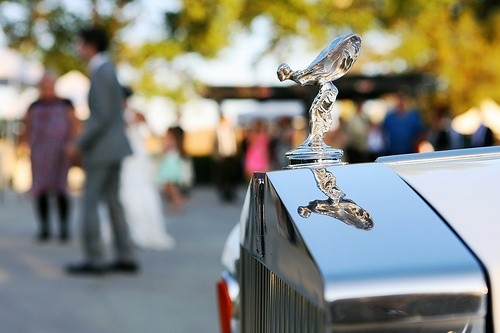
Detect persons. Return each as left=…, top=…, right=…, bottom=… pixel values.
left=161, top=21, right=498, bottom=212
left=277, top=32, right=361, bottom=149
left=16, top=75, right=80, bottom=243
left=68, top=21, right=140, bottom=277
left=111, top=86, right=177, bottom=250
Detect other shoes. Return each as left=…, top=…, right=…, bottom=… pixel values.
left=68, top=262, right=103, bottom=273
left=41, top=229, right=49, bottom=239
left=103, top=261, right=137, bottom=271
left=61, top=228, right=68, bottom=239
